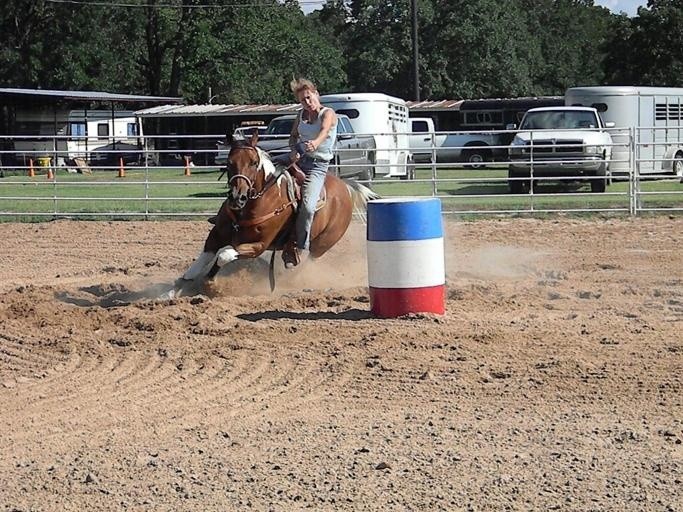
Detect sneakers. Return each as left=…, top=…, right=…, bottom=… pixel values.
left=280, top=245, right=297, bottom=269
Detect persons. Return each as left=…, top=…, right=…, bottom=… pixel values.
left=205, top=76, right=338, bottom=270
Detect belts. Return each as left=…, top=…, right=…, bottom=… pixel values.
left=302, top=154, right=325, bottom=162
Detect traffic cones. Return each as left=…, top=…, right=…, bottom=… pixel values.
left=29, top=159, right=35, bottom=176
left=119, top=157, right=125, bottom=177
left=185, top=158, right=190, bottom=175
left=48, top=162, right=53, bottom=178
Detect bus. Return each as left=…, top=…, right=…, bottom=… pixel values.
left=11, top=107, right=144, bottom=170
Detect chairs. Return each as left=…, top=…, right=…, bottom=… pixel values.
left=575, top=119, right=592, bottom=128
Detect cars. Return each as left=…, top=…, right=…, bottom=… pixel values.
left=508, top=106, right=614, bottom=194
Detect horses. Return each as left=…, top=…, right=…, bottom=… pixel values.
left=160, top=127, right=382, bottom=298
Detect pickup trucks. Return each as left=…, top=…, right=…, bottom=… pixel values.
left=214, top=113, right=377, bottom=189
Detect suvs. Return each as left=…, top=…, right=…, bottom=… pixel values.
left=407, top=117, right=507, bottom=169
